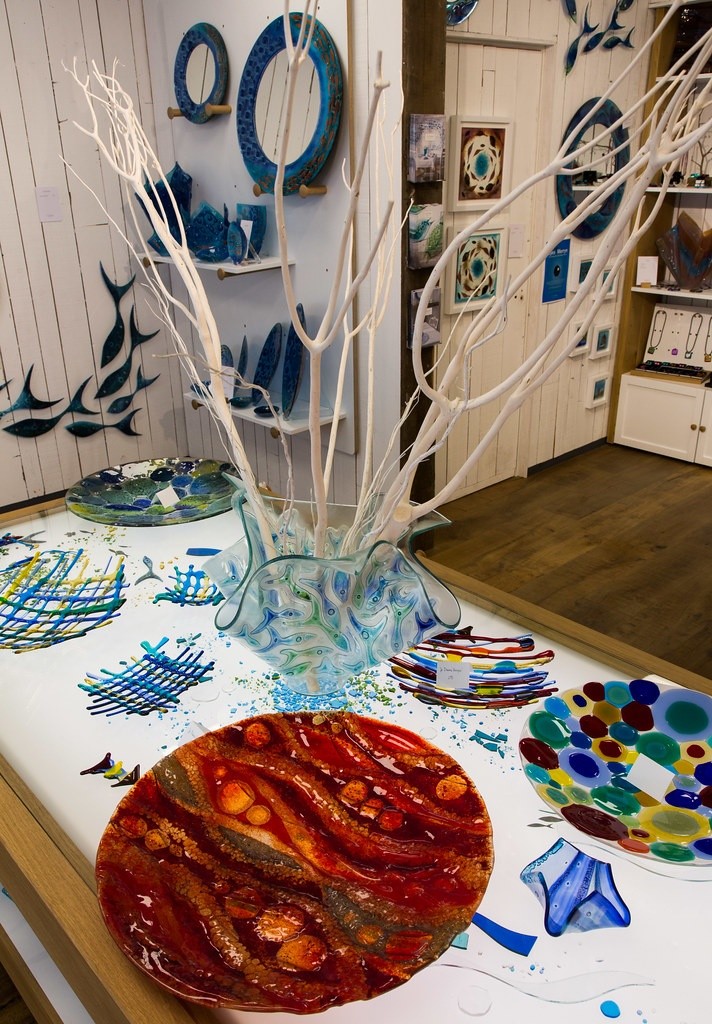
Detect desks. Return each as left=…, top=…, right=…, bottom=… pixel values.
left=0, top=494, right=712, bottom=1024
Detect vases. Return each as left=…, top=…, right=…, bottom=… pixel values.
left=205, top=492, right=460, bottom=698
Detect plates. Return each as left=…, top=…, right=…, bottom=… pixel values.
left=64, top=456, right=259, bottom=528
left=190, top=381, right=210, bottom=396
left=254, top=405, right=279, bottom=416
left=220, top=344, right=233, bottom=367
left=518, top=679, right=712, bottom=870
left=230, top=396, right=251, bottom=407
left=251, top=323, right=282, bottom=405
left=94, top=710, right=496, bottom=1015
left=280, top=304, right=308, bottom=419
left=232, top=336, right=249, bottom=392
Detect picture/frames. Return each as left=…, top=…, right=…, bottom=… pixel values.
left=591, top=264, right=620, bottom=302
left=440, top=224, right=512, bottom=316
left=586, top=372, right=611, bottom=409
left=565, top=318, right=594, bottom=359
left=590, top=321, right=617, bottom=360
left=446, top=114, right=517, bottom=214
left=570, top=254, right=597, bottom=294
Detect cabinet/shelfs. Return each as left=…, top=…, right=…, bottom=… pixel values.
left=128, top=229, right=348, bottom=435
left=605, top=0, right=712, bottom=468
